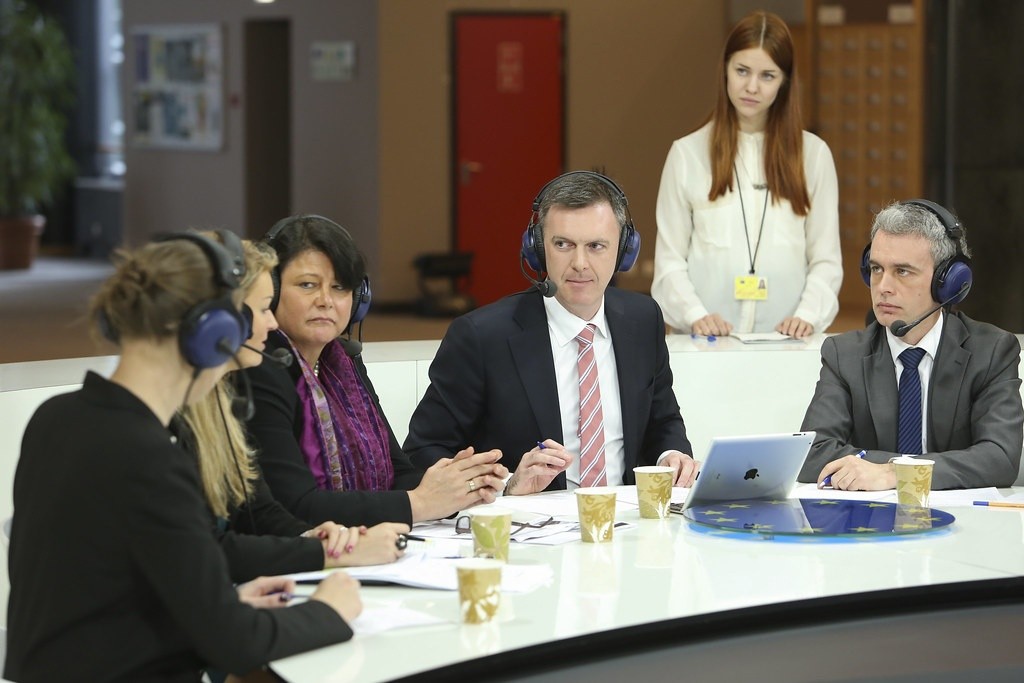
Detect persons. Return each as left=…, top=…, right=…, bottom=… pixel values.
left=227, top=212, right=508, bottom=530
left=799, top=197, right=1024, bottom=491
left=0, top=230, right=360, bottom=683
left=402, top=167, right=698, bottom=497
left=182, top=230, right=410, bottom=584
left=650, top=12, right=844, bottom=341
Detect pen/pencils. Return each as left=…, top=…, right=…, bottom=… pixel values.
left=403, top=534, right=426, bottom=542
left=973, top=501, right=1024, bottom=508
left=693, top=335, right=716, bottom=341
left=537, top=441, right=545, bottom=449
left=818, top=450, right=867, bottom=490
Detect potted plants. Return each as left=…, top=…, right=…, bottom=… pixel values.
left=0, top=0, right=72, bottom=268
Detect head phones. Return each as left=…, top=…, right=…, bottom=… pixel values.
left=261, top=213, right=372, bottom=324
left=861, top=199, right=974, bottom=306
left=522, top=170, right=641, bottom=272
left=157, top=228, right=253, bottom=369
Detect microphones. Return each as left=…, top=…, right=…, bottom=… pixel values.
left=344, top=320, right=364, bottom=360
left=223, top=343, right=253, bottom=420
left=520, top=258, right=557, bottom=297
left=891, top=285, right=969, bottom=337
left=241, top=343, right=292, bottom=369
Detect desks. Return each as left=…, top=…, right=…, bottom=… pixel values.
left=246, top=475, right=1024, bottom=683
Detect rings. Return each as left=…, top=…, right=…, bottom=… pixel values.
left=395, top=538, right=408, bottom=550
left=469, top=480, right=475, bottom=492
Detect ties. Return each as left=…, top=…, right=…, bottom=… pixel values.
left=575, top=324, right=608, bottom=489
left=897, top=348, right=926, bottom=454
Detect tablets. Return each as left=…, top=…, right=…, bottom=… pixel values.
left=680, top=431, right=816, bottom=512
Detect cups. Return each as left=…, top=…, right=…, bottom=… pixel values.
left=632, top=466, right=676, bottom=520
left=893, top=459, right=935, bottom=507
left=455, top=558, right=504, bottom=624
left=894, top=503, right=932, bottom=532
left=574, top=487, right=618, bottom=542
left=466, top=507, right=515, bottom=564
